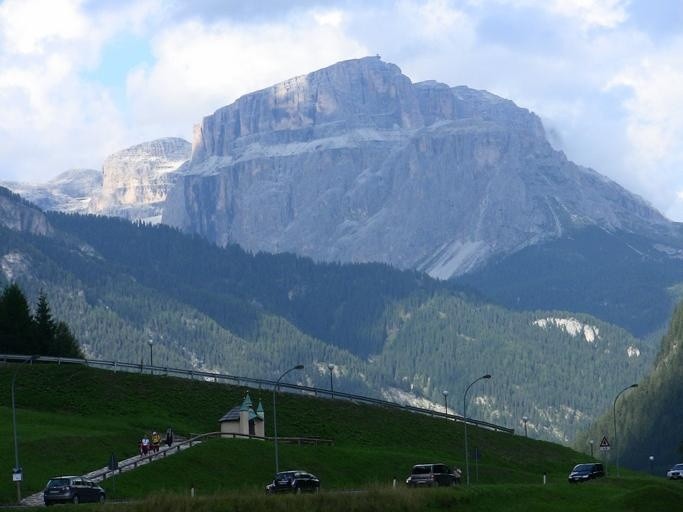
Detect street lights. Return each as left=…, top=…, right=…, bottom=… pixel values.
left=463, top=374, right=492, bottom=485
left=273, top=364, right=305, bottom=472
left=522, top=416, right=529, bottom=437
left=148, top=338, right=154, bottom=375
left=648, top=455, right=655, bottom=475
left=11, top=354, right=40, bottom=506
left=328, top=363, right=335, bottom=398
left=442, top=390, right=449, bottom=419
left=614, top=383, right=639, bottom=477
left=589, top=439, right=594, bottom=457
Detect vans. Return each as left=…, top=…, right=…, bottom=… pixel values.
left=406, top=463, right=457, bottom=487
left=568, top=463, right=604, bottom=483
left=265, top=470, right=320, bottom=494
left=43, top=475, right=106, bottom=505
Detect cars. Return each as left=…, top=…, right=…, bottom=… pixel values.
left=667, top=464, right=683, bottom=479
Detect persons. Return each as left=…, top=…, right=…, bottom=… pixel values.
left=453, top=466, right=462, bottom=485
left=138, top=426, right=174, bottom=458
